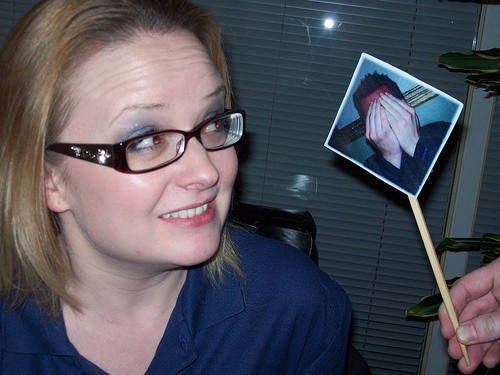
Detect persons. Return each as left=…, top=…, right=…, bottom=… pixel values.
left=0, top=1, right=355, bottom=374
left=439, top=258, right=500, bottom=375
left=350, top=70, right=451, bottom=195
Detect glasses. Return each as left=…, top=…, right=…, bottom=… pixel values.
left=45, top=109, right=246, bottom=174
